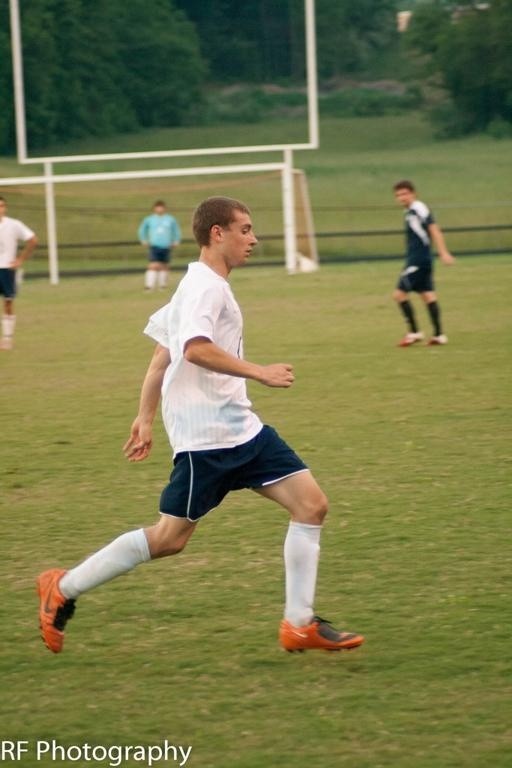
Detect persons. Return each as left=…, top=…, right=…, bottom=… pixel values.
left=138, top=200, right=180, bottom=292
left=382, top=175, right=456, bottom=351
left=37, top=198, right=364, bottom=656
left=1, top=197, right=40, bottom=354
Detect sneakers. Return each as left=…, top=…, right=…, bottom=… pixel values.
left=278, top=611, right=364, bottom=657
left=394, top=327, right=425, bottom=350
left=423, top=333, right=449, bottom=345
left=143, top=286, right=167, bottom=293
left=34, top=567, right=70, bottom=653
left=0, top=334, right=13, bottom=352
